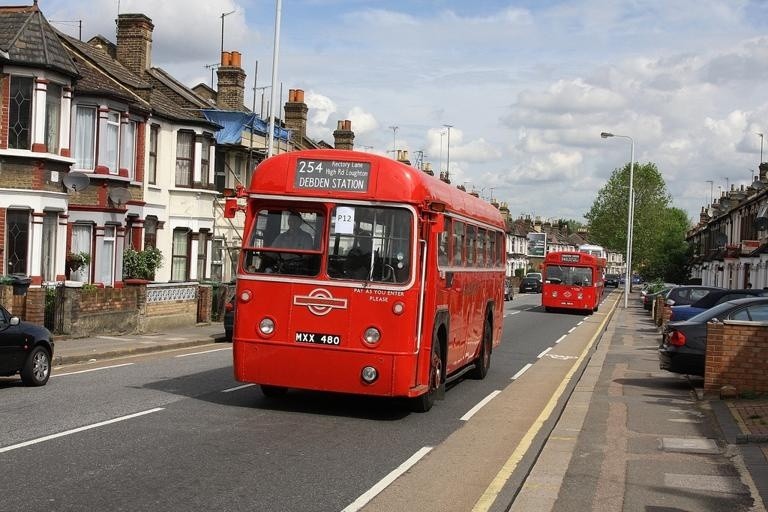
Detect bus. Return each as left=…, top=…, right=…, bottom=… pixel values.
left=538, top=244, right=606, bottom=316
left=221, top=149, right=508, bottom=413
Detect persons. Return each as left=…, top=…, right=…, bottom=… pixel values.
left=264, top=212, right=315, bottom=276
left=342, top=231, right=381, bottom=282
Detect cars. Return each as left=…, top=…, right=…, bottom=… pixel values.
left=0, top=304, right=54, bottom=386
left=638, top=280, right=768, bottom=378
left=604, top=272, right=643, bottom=288
left=504, top=279, right=515, bottom=301
left=223, top=294, right=235, bottom=338
left=519, top=272, right=542, bottom=293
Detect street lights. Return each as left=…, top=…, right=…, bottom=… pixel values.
left=620, top=186, right=635, bottom=293
left=600, top=131, right=635, bottom=308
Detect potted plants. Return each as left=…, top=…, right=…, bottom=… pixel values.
left=122, top=241, right=163, bottom=284
